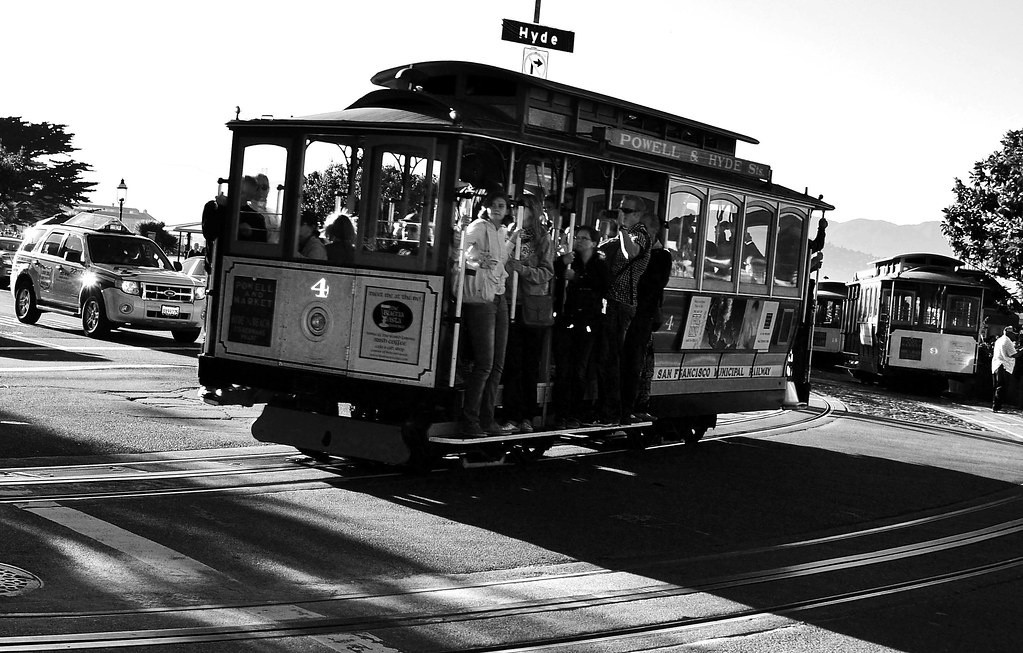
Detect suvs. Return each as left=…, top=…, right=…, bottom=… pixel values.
left=9, top=223, right=206, bottom=344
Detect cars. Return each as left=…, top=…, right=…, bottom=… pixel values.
left=0, top=237, right=23, bottom=289
left=180, top=255, right=209, bottom=285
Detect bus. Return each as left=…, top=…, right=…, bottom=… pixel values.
left=811, top=252, right=1022, bottom=405
left=172, top=222, right=207, bottom=262
left=195, top=59, right=834, bottom=468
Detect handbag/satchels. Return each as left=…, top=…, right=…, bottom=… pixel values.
left=522, top=295, right=555, bottom=326
left=450, top=219, right=500, bottom=304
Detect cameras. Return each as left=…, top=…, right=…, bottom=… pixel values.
left=604, top=210, right=619, bottom=219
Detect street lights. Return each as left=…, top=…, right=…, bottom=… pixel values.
left=117, top=178, right=128, bottom=221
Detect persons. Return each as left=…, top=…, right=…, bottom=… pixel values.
left=992, top=326, right=1023, bottom=413
left=456, top=190, right=672, bottom=436
left=195, top=174, right=433, bottom=410
left=772, top=217, right=828, bottom=282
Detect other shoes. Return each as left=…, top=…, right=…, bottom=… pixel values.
left=501, top=420, right=521, bottom=432
left=629, top=413, right=643, bottom=423
left=482, top=423, right=512, bottom=435
left=641, top=412, right=658, bottom=421
left=521, top=419, right=534, bottom=432
left=546, top=414, right=621, bottom=430
left=459, top=429, right=488, bottom=437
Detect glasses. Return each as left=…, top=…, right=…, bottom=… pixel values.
left=619, top=207, right=641, bottom=214
left=510, top=203, right=530, bottom=209
left=575, top=236, right=591, bottom=241
left=261, top=184, right=269, bottom=190
